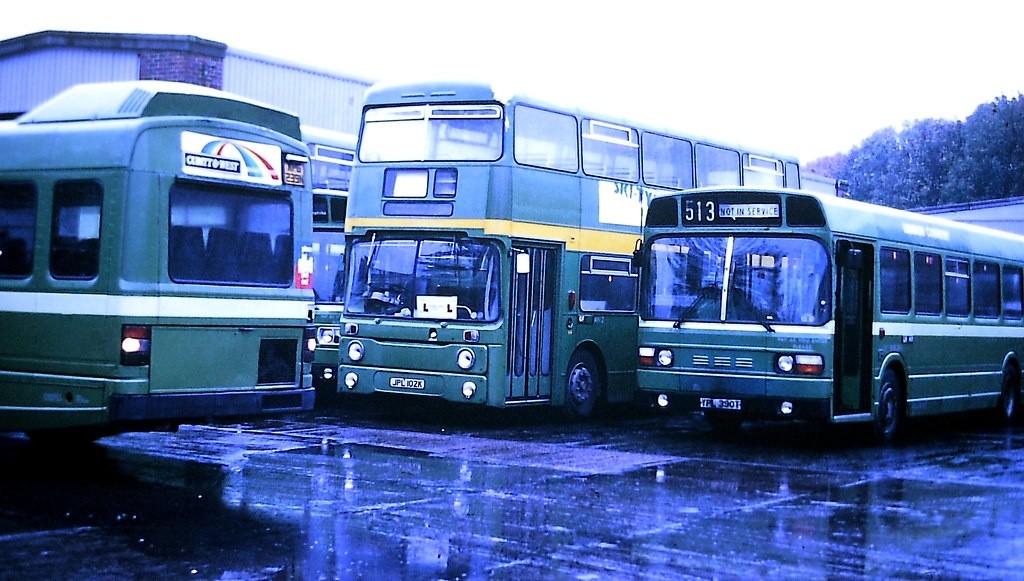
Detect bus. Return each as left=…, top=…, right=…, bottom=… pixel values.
left=630, top=186, right=1024, bottom=440
left=0, top=80, right=316, bottom=446
left=311, top=189, right=352, bottom=378
left=339, top=79, right=801, bottom=417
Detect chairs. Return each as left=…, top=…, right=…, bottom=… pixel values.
left=432, top=279, right=499, bottom=322
left=4, top=227, right=292, bottom=286
